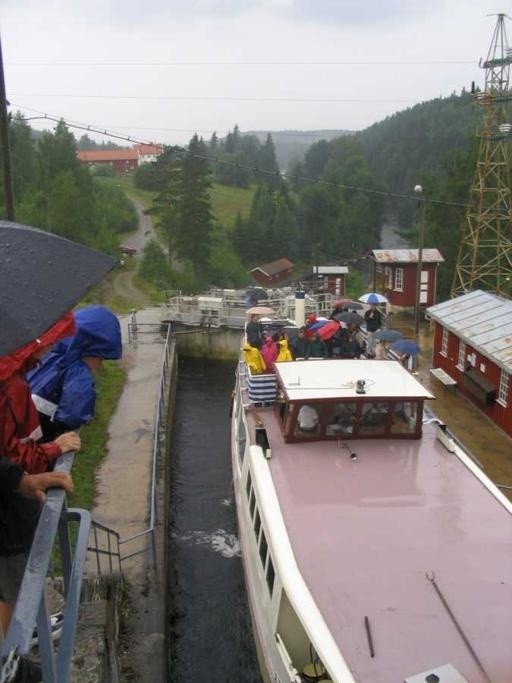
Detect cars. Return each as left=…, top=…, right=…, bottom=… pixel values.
left=117, top=245, right=138, bottom=258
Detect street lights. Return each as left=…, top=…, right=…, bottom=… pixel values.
left=411, top=183, right=425, bottom=334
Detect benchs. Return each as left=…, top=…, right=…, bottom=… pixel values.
left=429, top=367, right=457, bottom=395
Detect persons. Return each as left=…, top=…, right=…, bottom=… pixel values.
left=243, top=314, right=409, bottom=375
left=1, top=304, right=123, bottom=502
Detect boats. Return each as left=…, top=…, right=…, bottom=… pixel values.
left=226, top=277, right=511, bottom=682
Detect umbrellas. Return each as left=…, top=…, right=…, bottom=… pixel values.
left=244, top=293, right=419, bottom=361
left=2, top=221, right=120, bottom=358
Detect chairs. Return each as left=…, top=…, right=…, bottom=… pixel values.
left=314, top=658, right=333, bottom=683
left=301, top=643, right=328, bottom=683
